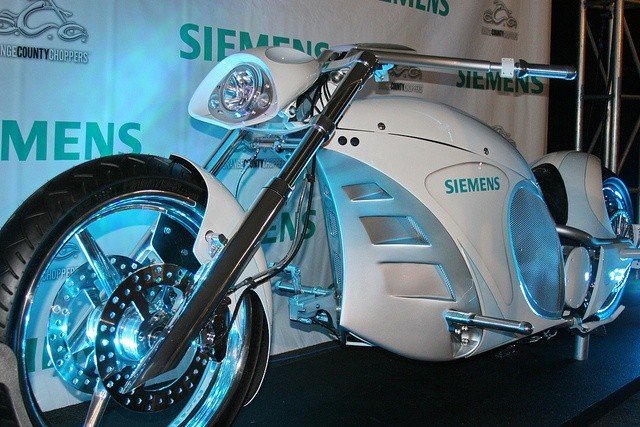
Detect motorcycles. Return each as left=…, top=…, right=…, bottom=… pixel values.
left=0, top=43, right=639, bottom=427
